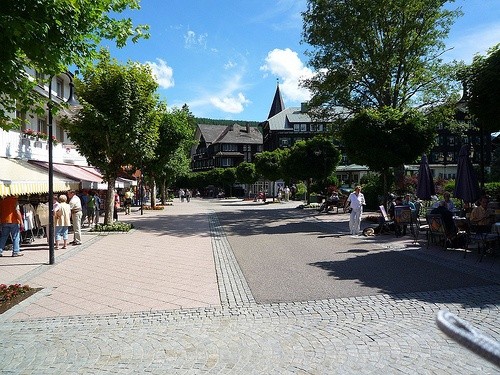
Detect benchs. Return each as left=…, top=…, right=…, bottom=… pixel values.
left=329, top=197, right=348, bottom=214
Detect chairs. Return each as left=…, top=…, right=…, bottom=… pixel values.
left=379, top=196, right=500, bottom=262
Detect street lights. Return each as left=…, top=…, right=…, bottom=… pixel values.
left=48, top=72, right=84, bottom=265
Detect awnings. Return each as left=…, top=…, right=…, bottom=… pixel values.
left=28, top=160, right=104, bottom=183
left=0, top=158, right=79, bottom=198
left=82, top=167, right=137, bottom=185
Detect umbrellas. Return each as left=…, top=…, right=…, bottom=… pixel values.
left=454, top=143, right=483, bottom=208
left=417, top=153, right=434, bottom=206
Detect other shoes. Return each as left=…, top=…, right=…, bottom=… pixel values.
left=62, top=246, right=66, bottom=249
left=349, top=230, right=353, bottom=234
left=70, top=241, right=76, bottom=244
left=12, top=253, right=24, bottom=256
left=355, top=233, right=361, bottom=235
left=55, top=247, right=59, bottom=250
left=73, top=243, right=81, bottom=246
left=125, top=213, right=127, bottom=215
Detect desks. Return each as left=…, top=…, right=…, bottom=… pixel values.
left=491, top=222, right=500, bottom=252
left=451, top=216, right=467, bottom=232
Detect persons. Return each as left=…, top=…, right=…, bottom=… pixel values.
left=132, top=186, right=146, bottom=206
left=125, top=188, right=132, bottom=215
left=67, top=189, right=82, bottom=245
left=79, top=188, right=100, bottom=226
left=253, top=192, right=265, bottom=202
left=344, top=186, right=366, bottom=235
left=178, top=188, right=192, bottom=202
left=46, top=194, right=71, bottom=250
left=113, top=189, right=119, bottom=222
left=277, top=184, right=297, bottom=201
left=470, top=196, right=500, bottom=256
left=0, top=195, right=24, bottom=257
left=390, top=195, right=416, bottom=220
left=430, top=201, right=455, bottom=234
left=318, top=191, right=339, bottom=212
left=432, top=192, right=453, bottom=210
